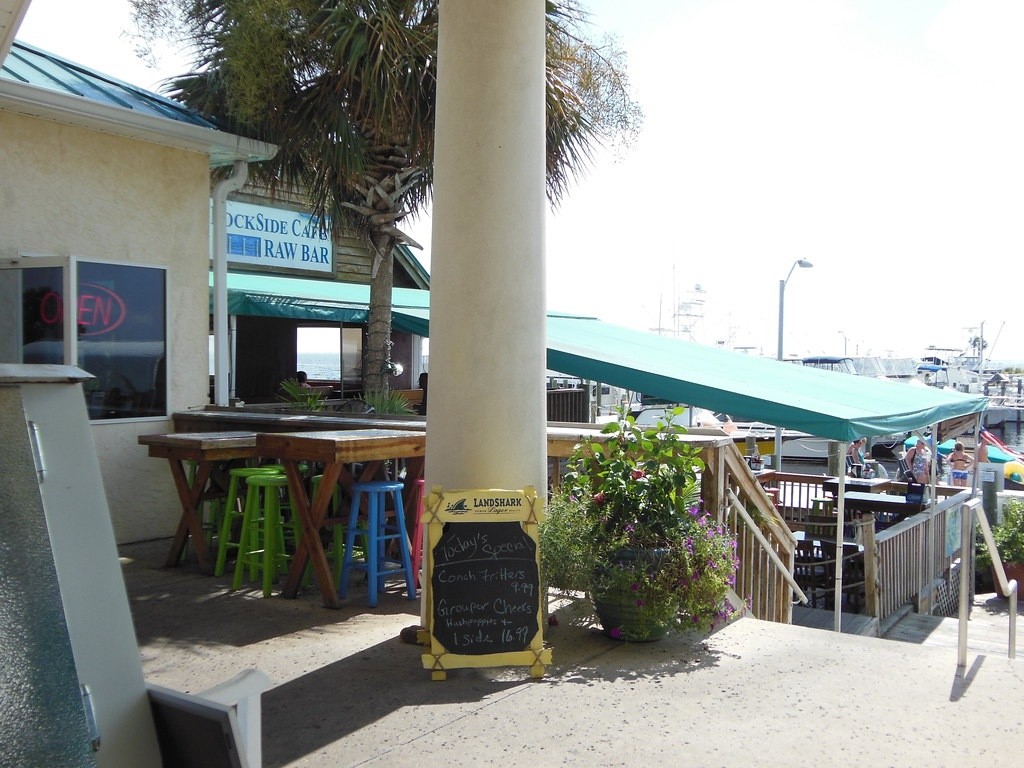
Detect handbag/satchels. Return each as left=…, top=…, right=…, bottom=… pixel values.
left=898, top=458, right=909, bottom=473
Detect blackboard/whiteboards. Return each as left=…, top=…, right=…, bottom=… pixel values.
left=415, top=484, right=555, bottom=682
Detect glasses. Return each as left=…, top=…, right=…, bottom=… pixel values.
left=920, top=442, right=924, bottom=445
left=860, top=442, right=863, bottom=444
left=862, top=440, right=865, bottom=443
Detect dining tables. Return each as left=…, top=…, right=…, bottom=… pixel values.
left=791, top=530, right=864, bottom=563
left=833, top=491, right=937, bottom=539
left=257, top=429, right=426, bottom=610
left=138, top=433, right=262, bottom=577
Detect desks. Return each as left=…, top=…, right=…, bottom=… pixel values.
left=823, top=479, right=891, bottom=495
left=728, top=468, right=777, bottom=490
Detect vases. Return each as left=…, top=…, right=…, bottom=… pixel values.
left=591, top=550, right=680, bottom=643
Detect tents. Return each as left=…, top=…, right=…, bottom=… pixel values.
left=210, top=270, right=989, bottom=633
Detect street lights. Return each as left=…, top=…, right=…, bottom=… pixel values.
left=774, top=260, right=812, bottom=503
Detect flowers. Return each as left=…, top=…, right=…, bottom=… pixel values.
left=539, top=402, right=752, bottom=639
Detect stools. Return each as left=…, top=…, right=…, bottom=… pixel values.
left=765, top=486, right=780, bottom=511
left=181, top=459, right=425, bottom=608
left=810, top=498, right=833, bottom=535
left=766, top=492, right=774, bottom=504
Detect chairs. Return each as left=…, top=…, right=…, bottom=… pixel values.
left=791, top=516, right=864, bottom=614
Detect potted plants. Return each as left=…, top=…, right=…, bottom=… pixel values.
left=975, top=496, right=1024, bottom=603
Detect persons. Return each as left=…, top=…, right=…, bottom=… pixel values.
left=946, top=442, right=974, bottom=486
left=846, top=437, right=870, bottom=465
left=297, top=371, right=311, bottom=389
left=978, top=436, right=989, bottom=462
left=905, top=440, right=932, bottom=484
